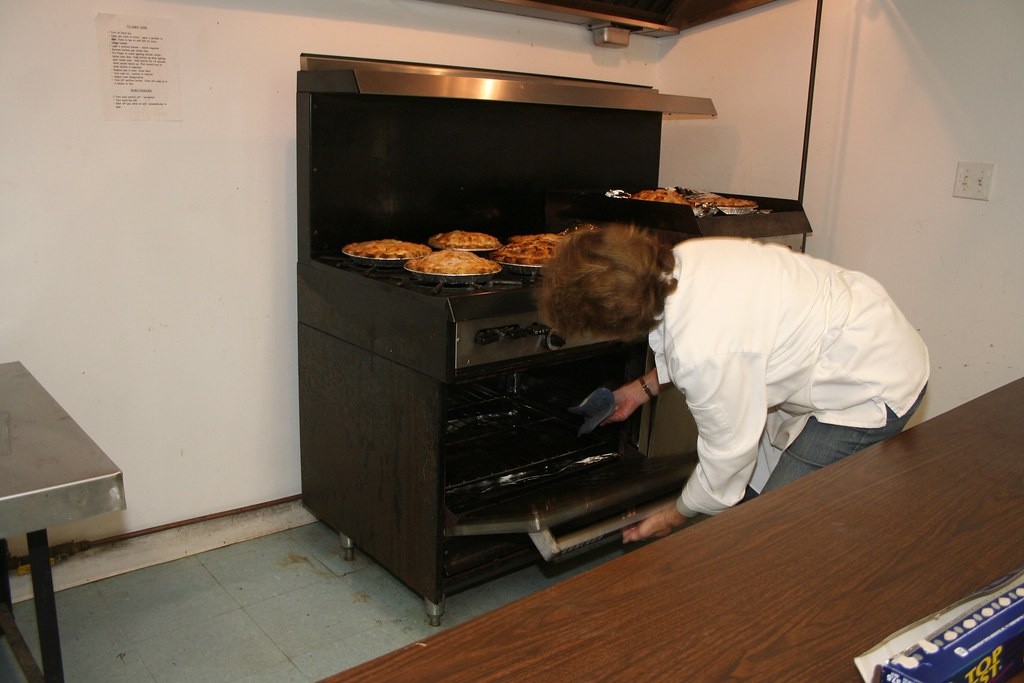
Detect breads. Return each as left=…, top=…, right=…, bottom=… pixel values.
left=342, top=229, right=563, bottom=276
left=630, top=188, right=758, bottom=215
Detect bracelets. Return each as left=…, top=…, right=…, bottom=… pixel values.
left=639, top=376, right=656, bottom=404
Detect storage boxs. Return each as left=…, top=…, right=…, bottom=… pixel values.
left=853, top=567, right=1023, bottom=683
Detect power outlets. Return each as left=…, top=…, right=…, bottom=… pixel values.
left=953, top=162, right=996, bottom=200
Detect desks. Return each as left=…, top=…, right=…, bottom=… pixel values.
left=317, top=377, right=1024, bottom=682
left=0, top=362, right=127, bottom=683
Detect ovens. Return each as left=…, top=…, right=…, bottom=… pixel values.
left=432, top=300, right=717, bottom=599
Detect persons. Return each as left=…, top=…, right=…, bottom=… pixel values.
left=539, top=223, right=930, bottom=543
